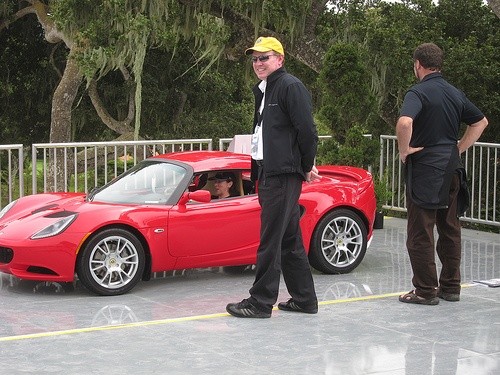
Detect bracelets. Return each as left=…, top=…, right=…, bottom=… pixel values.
left=190, top=200, right=192, bottom=202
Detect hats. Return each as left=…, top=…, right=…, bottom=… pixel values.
left=208, top=171, right=235, bottom=181
left=245, top=36, right=284, bottom=56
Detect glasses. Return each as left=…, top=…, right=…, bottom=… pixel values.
left=251, top=54, right=278, bottom=63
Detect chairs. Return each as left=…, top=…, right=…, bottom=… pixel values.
left=203, top=171, right=244, bottom=197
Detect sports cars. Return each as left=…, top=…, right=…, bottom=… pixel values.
left=0, top=151, right=384, bottom=296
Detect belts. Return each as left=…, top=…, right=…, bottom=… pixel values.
left=255, top=160, right=263, bottom=166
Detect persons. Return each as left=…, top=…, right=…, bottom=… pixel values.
left=190, top=172, right=237, bottom=202
left=396, top=41, right=488, bottom=305
left=225, top=37, right=322, bottom=318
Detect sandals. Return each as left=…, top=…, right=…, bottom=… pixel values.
left=399, top=290, right=439, bottom=305
left=436, top=287, right=459, bottom=301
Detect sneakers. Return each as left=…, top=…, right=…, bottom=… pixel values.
left=278, top=298, right=317, bottom=313
left=226, top=299, right=271, bottom=318
